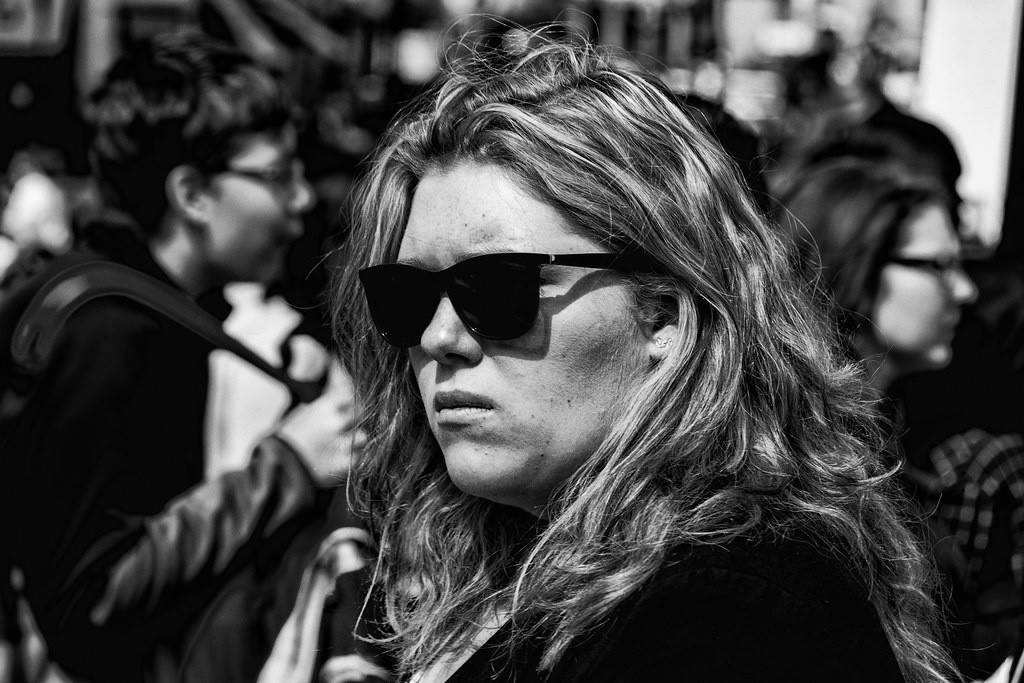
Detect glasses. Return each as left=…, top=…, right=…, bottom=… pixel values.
left=233, top=157, right=306, bottom=194
left=360, top=250, right=623, bottom=346
left=890, top=252, right=964, bottom=289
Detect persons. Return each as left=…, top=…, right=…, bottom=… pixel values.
left=1, top=18, right=1024, bottom=683
